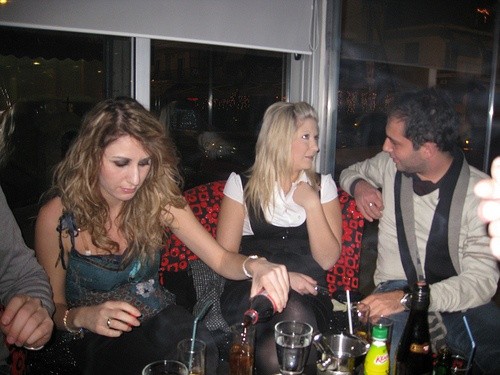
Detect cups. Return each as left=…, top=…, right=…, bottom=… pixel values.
left=451, top=354, right=473, bottom=375
left=229, top=326, right=256, bottom=375
left=273, top=320, right=313, bottom=374
left=142, top=338, right=206, bottom=375
left=345, top=302, right=393, bottom=375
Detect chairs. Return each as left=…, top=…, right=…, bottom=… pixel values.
left=159, top=184, right=365, bottom=297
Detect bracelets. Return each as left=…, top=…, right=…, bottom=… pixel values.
left=63, top=307, right=83, bottom=334
left=243, top=254, right=259, bottom=277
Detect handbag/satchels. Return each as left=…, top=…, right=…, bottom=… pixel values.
left=72, top=275, right=174, bottom=327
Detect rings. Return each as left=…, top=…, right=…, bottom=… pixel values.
left=107, top=318, right=112, bottom=326
left=368, top=202, right=374, bottom=208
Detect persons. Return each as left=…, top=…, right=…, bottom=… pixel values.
left=338, top=85, right=500, bottom=375
left=216, top=101, right=342, bottom=375
left=0, top=82, right=56, bottom=368
left=35, top=96, right=290, bottom=375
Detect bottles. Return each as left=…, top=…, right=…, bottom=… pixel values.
left=239, top=288, right=278, bottom=327
left=395, top=281, right=434, bottom=375
left=437, top=348, right=452, bottom=375
left=313, top=334, right=370, bottom=374
left=363, top=324, right=390, bottom=375
